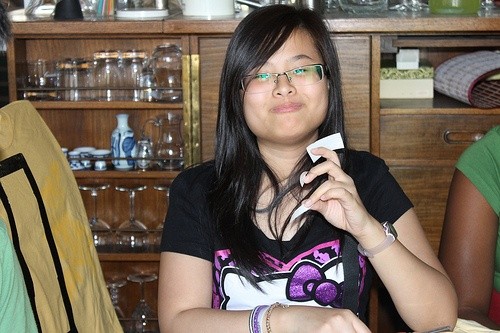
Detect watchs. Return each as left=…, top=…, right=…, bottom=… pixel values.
left=357, top=221, right=397, bottom=257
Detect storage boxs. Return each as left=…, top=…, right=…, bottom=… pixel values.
left=379, top=59, right=435, bottom=99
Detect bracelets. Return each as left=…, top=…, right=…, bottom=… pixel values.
left=249, top=302, right=283, bottom=332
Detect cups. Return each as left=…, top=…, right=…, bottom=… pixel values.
left=248, top=0, right=385, bottom=15
left=61, top=146, right=112, bottom=171
left=28, top=51, right=157, bottom=102
left=429, top=0, right=481, bottom=15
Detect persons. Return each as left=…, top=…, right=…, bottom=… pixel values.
left=159, top=4, right=457, bottom=333
left=439, top=124, right=498, bottom=333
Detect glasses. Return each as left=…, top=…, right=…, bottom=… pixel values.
left=240, top=64, right=328, bottom=94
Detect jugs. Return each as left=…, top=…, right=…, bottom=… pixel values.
left=141, top=113, right=185, bottom=170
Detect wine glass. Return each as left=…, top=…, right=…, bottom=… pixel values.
left=388, top=0, right=429, bottom=12
left=78, top=184, right=171, bottom=246
left=106, top=273, right=159, bottom=333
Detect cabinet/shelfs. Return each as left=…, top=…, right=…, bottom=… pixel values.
left=6, top=24, right=500, bottom=333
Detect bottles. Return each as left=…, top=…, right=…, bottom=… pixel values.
left=111, top=115, right=137, bottom=169
left=136, top=140, right=153, bottom=169
left=148, top=41, right=182, bottom=102
